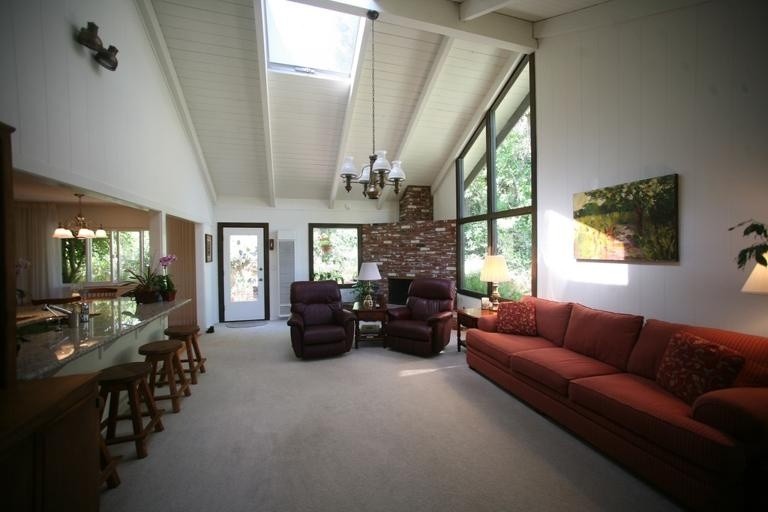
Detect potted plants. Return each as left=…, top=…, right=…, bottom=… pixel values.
left=119, top=263, right=173, bottom=304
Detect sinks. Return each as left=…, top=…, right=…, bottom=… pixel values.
left=17, top=314, right=70, bottom=334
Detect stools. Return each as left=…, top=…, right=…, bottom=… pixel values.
left=96, top=362, right=166, bottom=457
left=166, top=323, right=209, bottom=384
left=137, top=338, right=192, bottom=414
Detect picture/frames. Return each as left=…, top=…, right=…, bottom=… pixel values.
left=204, top=234, right=213, bottom=262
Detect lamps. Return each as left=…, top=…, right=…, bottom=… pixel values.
left=336, top=14, right=409, bottom=199
left=482, top=256, right=512, bottom=309
left=51, top=193, right=108, bottom=240
left=356, top=261, right=386, bottom=308
left=740, top=251, right=767, bottom=294
left=68, top=24, right=119, bottom=70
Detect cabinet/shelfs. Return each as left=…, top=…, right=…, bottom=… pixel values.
left=1, top=372, right=123, bottom=512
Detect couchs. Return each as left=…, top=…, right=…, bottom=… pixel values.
left=466, top=295, right=768, bottom=509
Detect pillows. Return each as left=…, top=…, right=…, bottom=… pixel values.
left=647, top=330, right=744, bottom=401
left=498, top=302, right=535, bottom=335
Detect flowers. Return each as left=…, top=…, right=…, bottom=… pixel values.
left=317, top=234, right=332, bottom=246
left=159, top=253, right=175, bottom=275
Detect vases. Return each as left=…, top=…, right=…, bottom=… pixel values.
left=162, top=290, right=177, bottom=300
left=322, top=246, right=331, bottom=253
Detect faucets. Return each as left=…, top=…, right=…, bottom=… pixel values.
left=41, top=303, right=61, bottom=328
left=43, top=304, right=72, bottom=317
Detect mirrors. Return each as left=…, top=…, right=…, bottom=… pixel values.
left=13, top=168, right=167, bottom=324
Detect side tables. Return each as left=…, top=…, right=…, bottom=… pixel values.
left=458, top=307, right=495, bottom=352
left=347, top=300, right=387, bottom=349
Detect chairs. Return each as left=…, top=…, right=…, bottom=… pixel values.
left=387, top=276, right=457, bottom=358
left=290, top=282, right=352, bottom=358
